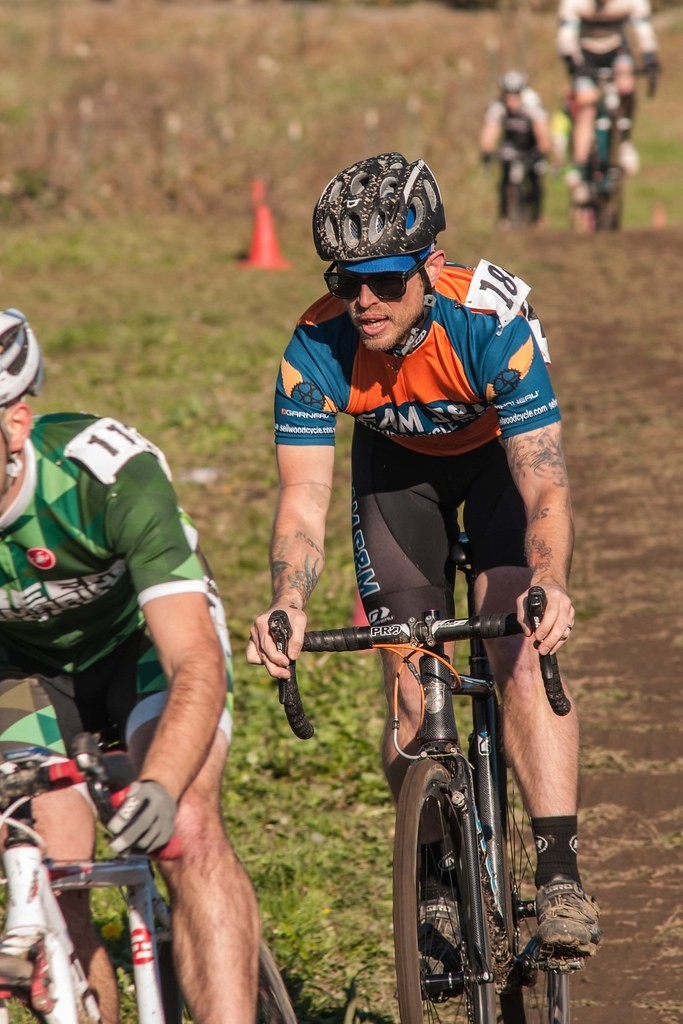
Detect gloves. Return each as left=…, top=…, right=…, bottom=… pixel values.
left=103, top=779, right=175, bottom=860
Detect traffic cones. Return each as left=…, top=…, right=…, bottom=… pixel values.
left=236, top=177, right=293, bottom=270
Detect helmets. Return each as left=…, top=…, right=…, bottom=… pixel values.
left=501, top=70, right=524, bottom=92
left=0, top=308, right=41, bottom=408
left=313, top=152, right=446, bottom=261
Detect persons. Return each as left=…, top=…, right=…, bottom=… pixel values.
left=0, top=307, right=261, bottom=1024
left=249, top=150, right=602, bottom=947
left=479, top=0, right=661, bottom=231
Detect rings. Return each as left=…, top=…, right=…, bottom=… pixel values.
left=567, top=624, right=572, bottom=630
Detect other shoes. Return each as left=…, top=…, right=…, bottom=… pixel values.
left=567, top=164, right=592, bottom=205
left=618, top=140, right=639, bottom=173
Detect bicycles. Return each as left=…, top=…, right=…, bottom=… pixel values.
left=268, top=586, right=571, bottom=1024
left=0, top=732, right=298, bottom=1024
left=479, top=54, right=662, bottom=233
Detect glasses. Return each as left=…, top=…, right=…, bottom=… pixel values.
left=324, top=251, right=434, bottom=299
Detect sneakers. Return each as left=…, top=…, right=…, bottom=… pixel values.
left=535, top=874, right=600, bottom=955
left=421, top=897, right=462, bottom=977
